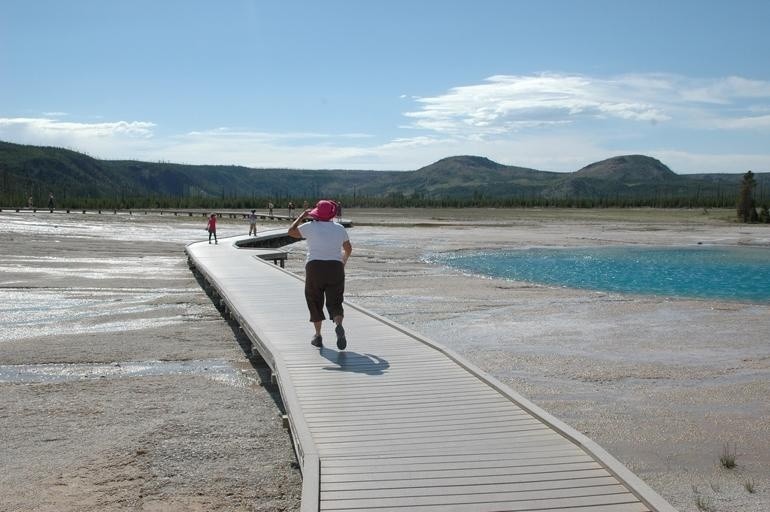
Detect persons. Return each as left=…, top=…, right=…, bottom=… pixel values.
left=303, top=201, right=309, bottom=217
left=288, top=200, right=353, bottom=349
left=268, top=200, right=274, bottom=216
left=288, top=202, right=293, bottom=217
left=206, top=212, right=219, bottom=245
left=248, top=209, right=258, bottom=237
left=336, top=201, right=343, bottom=223
left=291, top=201, right=296, bottom=218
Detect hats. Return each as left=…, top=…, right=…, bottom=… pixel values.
left=306, top=200, right=337, bottom=220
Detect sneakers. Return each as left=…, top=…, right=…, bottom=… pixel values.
left=336, top=324, right=346, bottom=349
left=310, top=335, right=322, bottom=347
